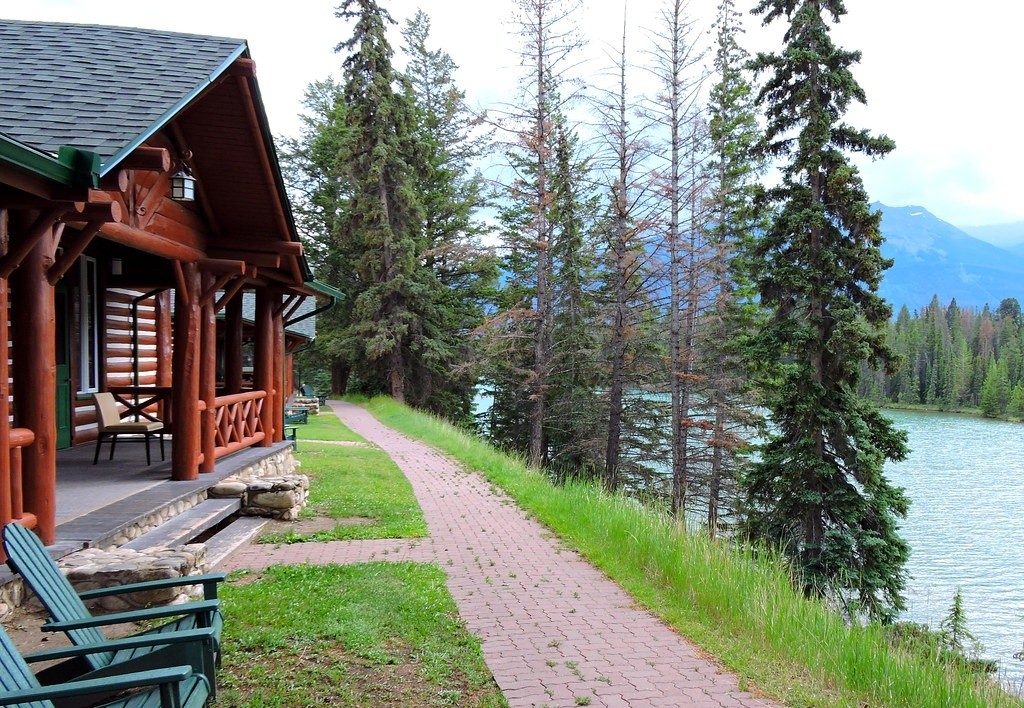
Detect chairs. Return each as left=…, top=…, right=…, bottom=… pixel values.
left=0, top=523, right=228, bottom=708
left=91, top=392, right=165, bottom=468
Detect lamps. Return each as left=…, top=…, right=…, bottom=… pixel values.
left=171, top=159, right=197, bottom=202
